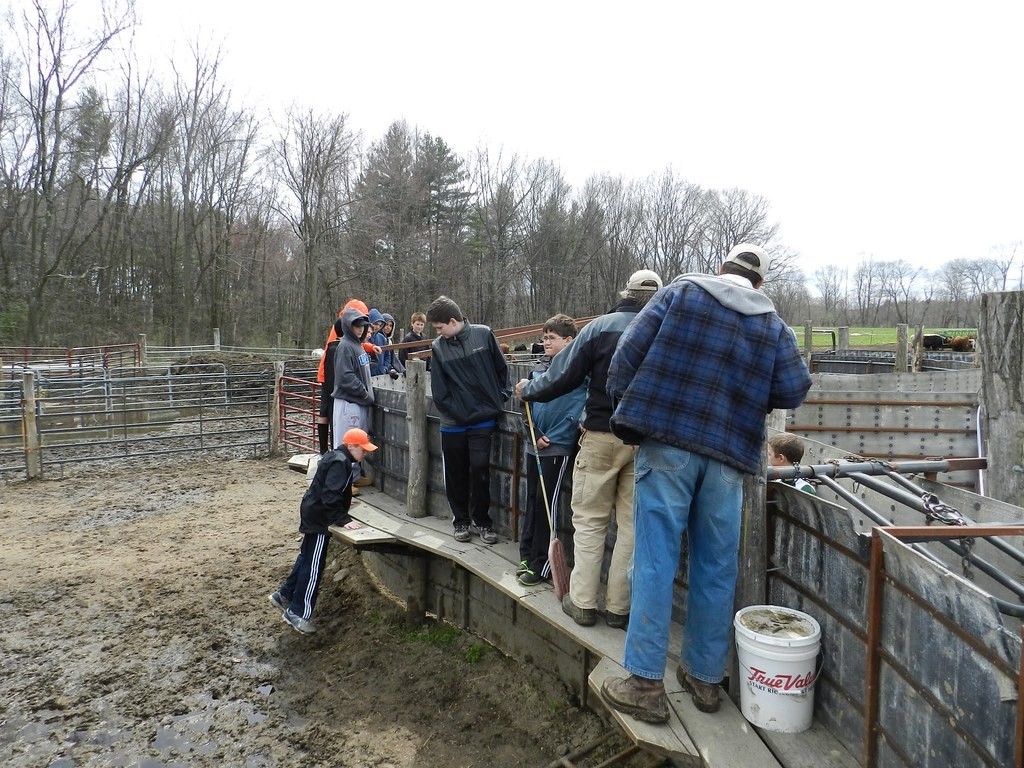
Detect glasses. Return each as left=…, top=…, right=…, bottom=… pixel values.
left=540, top=336, right=565, bottom=341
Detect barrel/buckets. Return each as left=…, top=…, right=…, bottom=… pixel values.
left=732, top=604, right=824, bottom=733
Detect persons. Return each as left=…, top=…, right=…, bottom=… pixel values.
left=515, top=314, right=589, bottom=586
left=381, top=313, right=406, bottom=380
left=324, top=318, right=344, bottom=450
left=331, top=306, right=374, bottom=496
left=601, top=244, right=812, bottom=723
left=316, top=299, right=382, bottom=455
left=425, top=295, right=513, bottom=543
left=398, top=312, right=431, bottom=371
left=768, top=432, right=817, bottom=496
left=364, top=323, right=374, bottom=341
left=516, top=269, right=663, bottom=628
left=368, top=309, right=391, bottom=376
left=268, top=427, right=378, bottom=633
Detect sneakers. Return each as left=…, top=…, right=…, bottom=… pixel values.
left=351, top=485, right=359, bottom=496
left=268, top=591, right=287, bottom=612
left=562, top=592, right=595, bottom=625
left=516, top=560, right=531, bottom=576
left=471, top=519, right=499, bottom=543
left=282, top=608, right=317, bottom=637
left=606, top=610, right=626, bottom=627
left=354, top=477, right=372, bottom=486
left=518, top=570, right=546, bottom=586
left=601, top=674, right=670, bottom=722
left=454, top=524, right=472, bottom=542
left=677, top=665, right=720, bottom=713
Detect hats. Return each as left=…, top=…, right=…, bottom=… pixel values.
left=352, top=317, right=370, bottom=327
left=627, top=270, right=664, bottom=291
left=724, top=243, right=770, bottom=279
left=343, top=428, right=378, bottom=452
